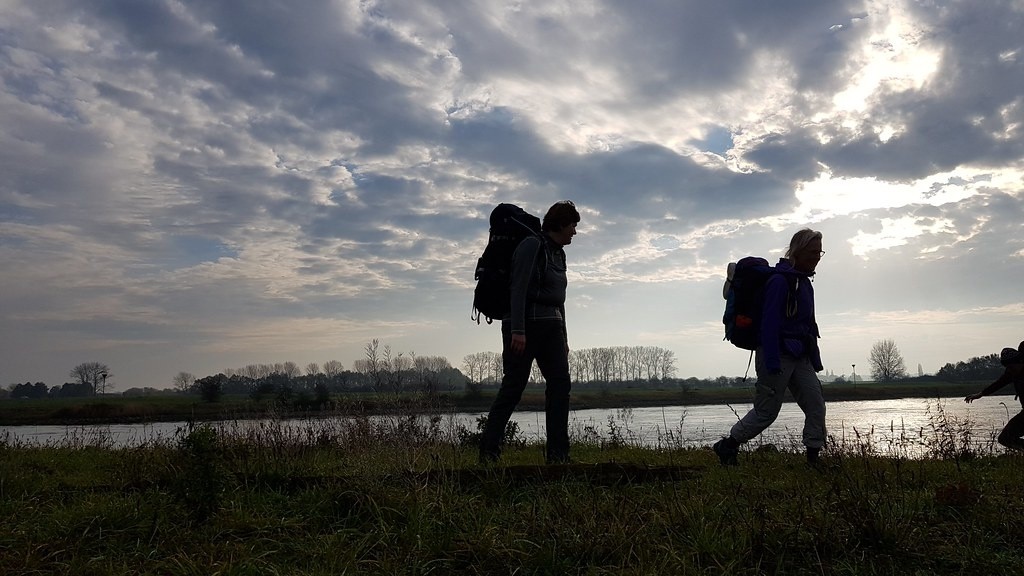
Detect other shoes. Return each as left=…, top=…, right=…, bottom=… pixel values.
left=804, top=458, right=842, bottom=476
left=713, top=441, right=741, bottom=468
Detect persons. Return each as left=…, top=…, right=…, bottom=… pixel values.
left=711, top=226, right=826, bottom=467
left=478, top=201, right=580, bottom=462
left=964, top=340, right=1024, bottom=449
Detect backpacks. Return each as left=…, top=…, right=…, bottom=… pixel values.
left=471, top=202, right=548, bottom=325
left=723, top=257, right=821, bottom=383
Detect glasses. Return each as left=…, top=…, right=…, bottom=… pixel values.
left=805, top=248, right=826, bottom=257
